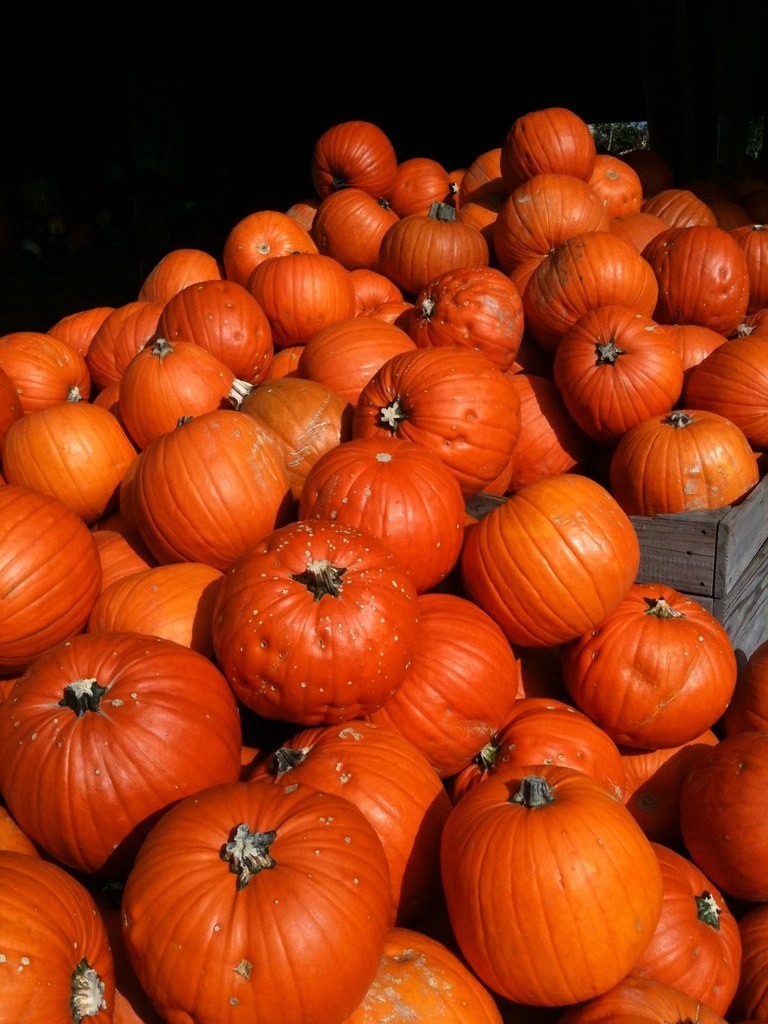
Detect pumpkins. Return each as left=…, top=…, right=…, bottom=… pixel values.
left=0, top=107, right=768, bottom=1024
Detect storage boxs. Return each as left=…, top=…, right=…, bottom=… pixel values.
left=465, top=467, right=768, bottom=687
